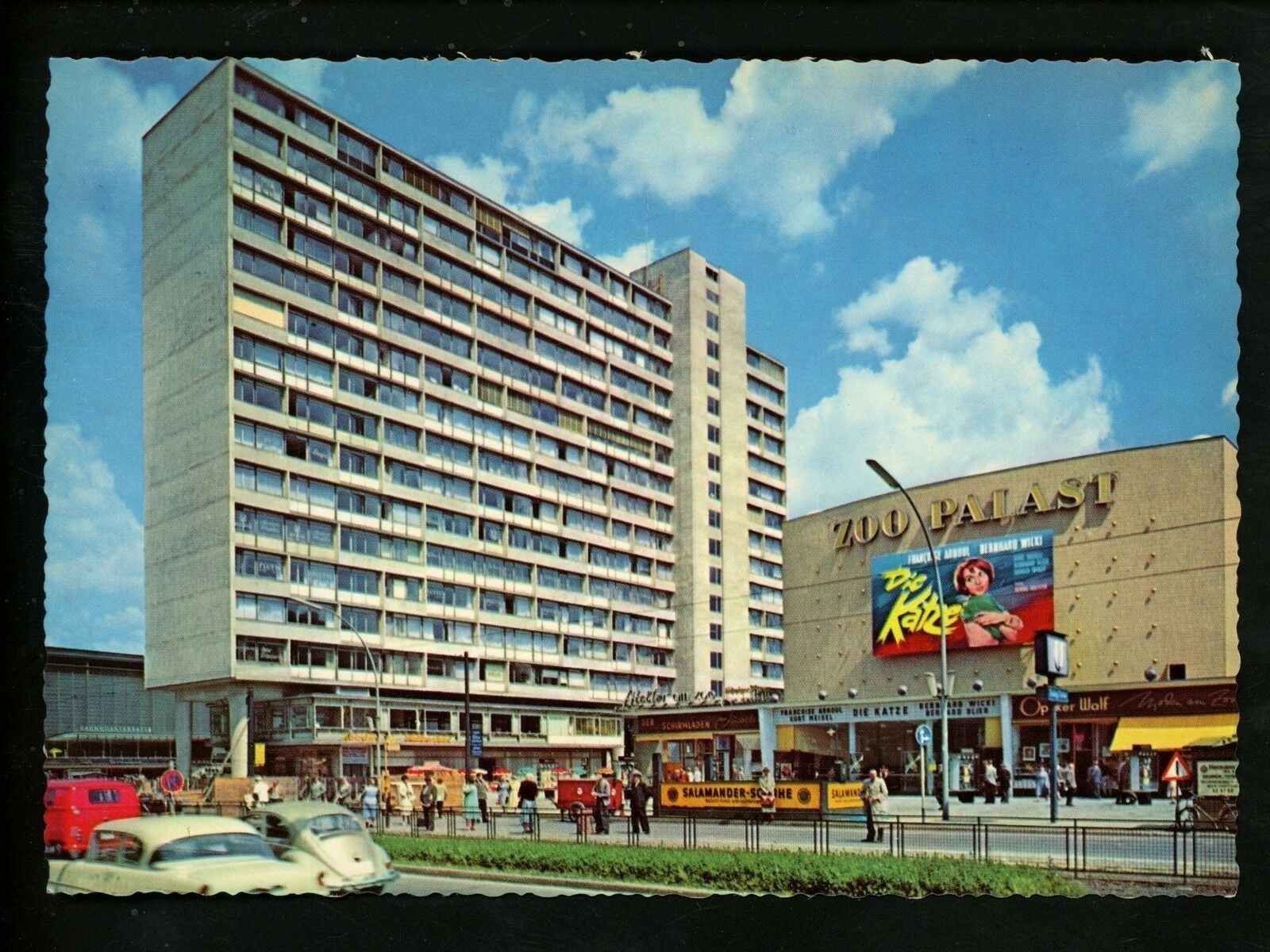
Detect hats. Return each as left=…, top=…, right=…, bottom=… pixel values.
left=630, top=769, right=642, bottom=777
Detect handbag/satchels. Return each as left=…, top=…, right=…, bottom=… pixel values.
left=761, top=794, right=775, bottom=807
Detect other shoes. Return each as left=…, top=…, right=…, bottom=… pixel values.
left=642, top=828, right=650, bottom=833
left=633, top=830, right=639, bottom=834
left=861, top=838, right=874, bottom=842
left=877, top=839, right=883, bottom=843
left=605, top=831, right=610, bottom=833
left=596, top=830, right=603, bottom=834
left=523, top=828, right=531, bottom=833
left=462, top=826, right=470, bottom=830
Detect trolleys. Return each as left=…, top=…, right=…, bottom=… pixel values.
left=547, top=779, right=624, bottom=824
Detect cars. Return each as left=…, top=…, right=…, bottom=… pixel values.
left=45, top=813, right=332, bottom=902
left=232, top=800, right=403, bottom=898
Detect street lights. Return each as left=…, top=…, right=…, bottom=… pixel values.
left=284, top=594, right=384, bottom=810
left=864, top=458, right=951, bottom=820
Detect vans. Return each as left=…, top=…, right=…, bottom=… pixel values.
left=43, top=780, right=143, bottom=857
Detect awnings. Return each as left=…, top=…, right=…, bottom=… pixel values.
left=1108, top=712, right=1239, bottom=753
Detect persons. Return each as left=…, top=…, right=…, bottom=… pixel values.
left=858, top=769, right=888, bottom=843
left=931, top=761, right=944, bottom=811
left=758, top=765, right=776, bottom=821
left=251, top=770, right=449, bottom=832
left=1032, top=755, right=1077, bottom=806
left=591, top=772, right=611, bottom=836
left=671, top=765, right=702, bottom=783
left=630, top=769, right=653, bottom=835
left=953, top=557, right=1025, bottom=647
left=1115, top=756, right=1129, bottom=805
left=517, top=772, right=538, bottom=834
left=462, top=772, right=512, bottom=831
left=1086, top=759, right=1102, bottom=800
left=983, top=757, right=1011, bottom=805
